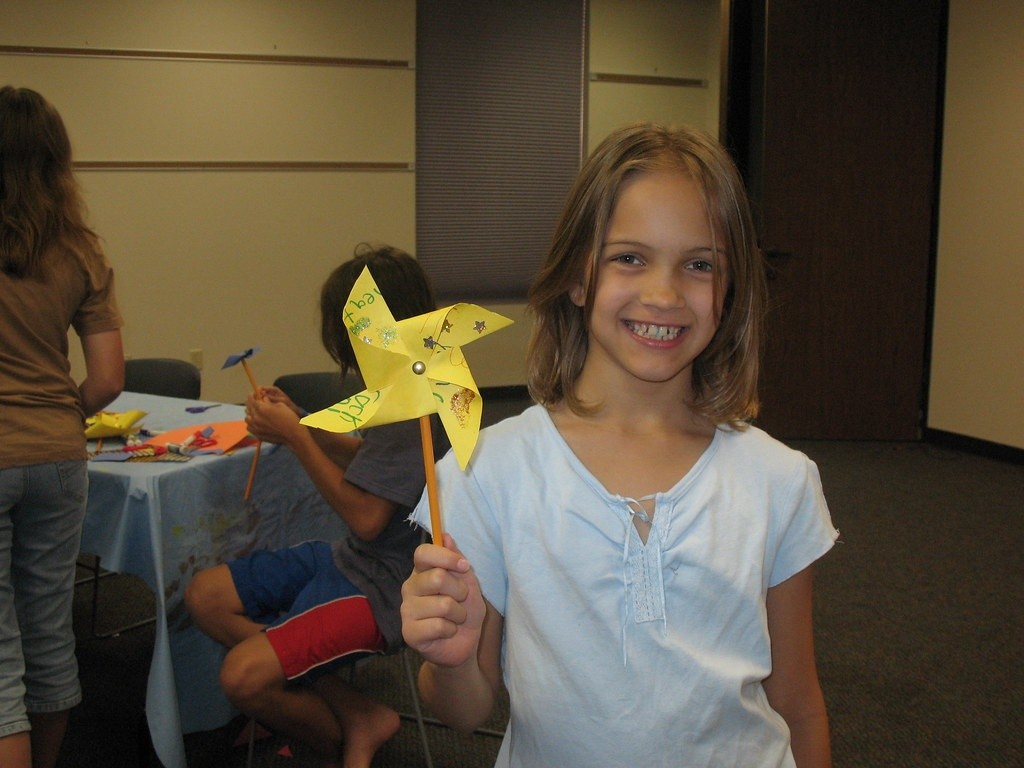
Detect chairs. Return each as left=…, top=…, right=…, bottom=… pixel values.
left=273, top=372, right=365, bottom=415
left=124, top=357, right=201, bottom=399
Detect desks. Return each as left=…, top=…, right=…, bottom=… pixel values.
left=78, top=390, right=431, bottom=767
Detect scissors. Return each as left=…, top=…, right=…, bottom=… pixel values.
left=186, top=404, right=221, bottom=413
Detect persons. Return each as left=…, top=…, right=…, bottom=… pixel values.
left=187, top=242, right=451, bottom=768
left=0, top=85, right=126, bottom=768
left=400, top=122, right=841, bottom=768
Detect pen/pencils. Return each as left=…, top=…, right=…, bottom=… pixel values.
left=94, top=438, right=103, bottom=454
left=140, top=429, right=164, bottom=436
